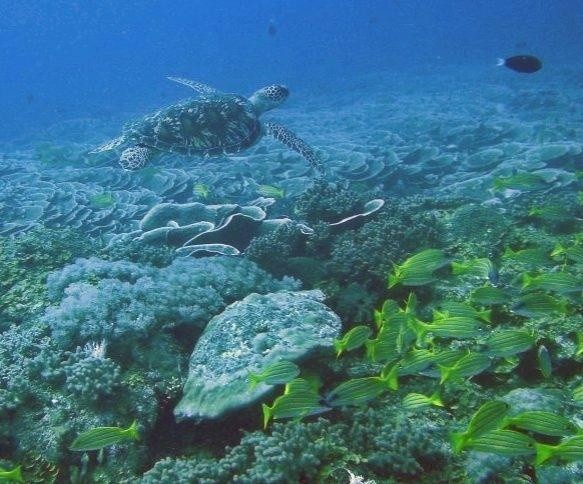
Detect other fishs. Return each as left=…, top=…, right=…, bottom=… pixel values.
left=365, top=239, right=583, bottom=467
